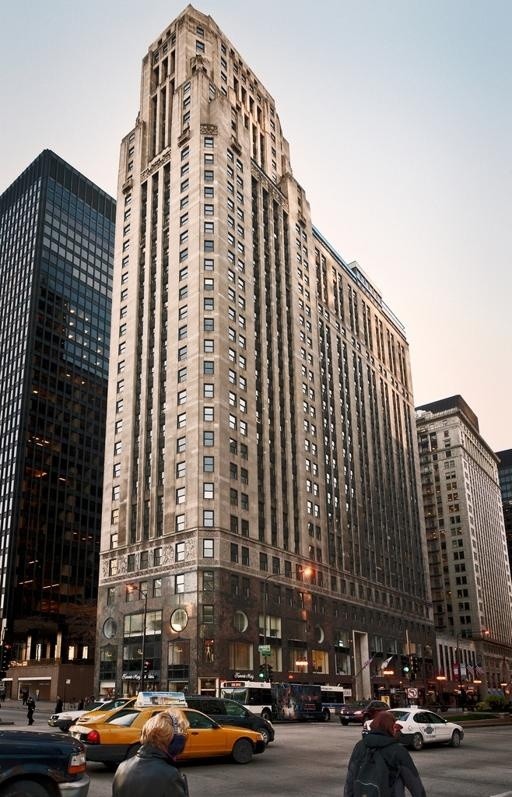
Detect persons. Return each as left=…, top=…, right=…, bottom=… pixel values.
left=77, top=695, right=95, bottom=711
left=343, top=711, right=427, bottom=797
left=111, top=710, right=189, bottom=797
left=54, top=696, right=63, bottom=714
left=26, top=697, right=36, bottom=726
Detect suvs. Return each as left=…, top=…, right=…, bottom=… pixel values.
left=340, top=696, right=391, bottom=725
left=0, top=724, right=94, bottom=796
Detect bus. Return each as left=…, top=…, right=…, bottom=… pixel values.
left=219, top=676, right=347, bottom=722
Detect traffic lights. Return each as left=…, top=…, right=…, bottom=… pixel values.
left=144, top=660, right=150, bottom=672
left=0, top=641, right=11, bottom=671
left=258, top=663, right=265, bottom=679
left=266, top=663, right=274, bottom=680
left=403, top=662, right=411, bottom=672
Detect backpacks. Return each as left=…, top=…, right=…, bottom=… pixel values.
left=351, top=747, right=398, bottom=797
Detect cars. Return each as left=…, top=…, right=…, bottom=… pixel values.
left=46, top=689, right=276, bottom=743
left=361, top=705, right=466, bottom=751
left=67, top=691, right=267, bottom=768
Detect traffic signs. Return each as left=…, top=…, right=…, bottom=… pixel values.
left=262, top=651, right=271, bottom=656
left=257, top=644, right=270, bottom=652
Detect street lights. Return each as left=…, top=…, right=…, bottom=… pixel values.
left=262, top=566, right=314, bottom=680
left=126, top=582, right=148, bottom=691
left=456, top=628, right=491, bottom=687
